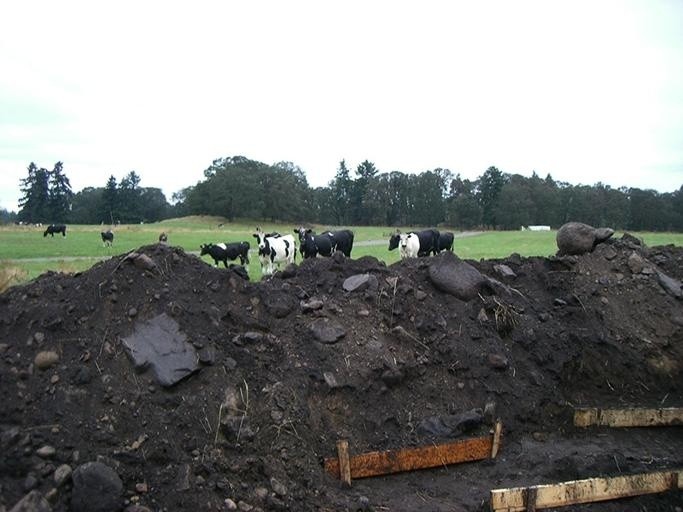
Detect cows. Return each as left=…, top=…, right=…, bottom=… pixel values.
left=253, top=227, right=354, bottom=277
left=101, top=231, right=113, bottom=247
left=200, top=241, right=251, bottom=273
left=44, top=225, right=66, bottom=238
left=389, top=229, right=456, bottom=260
